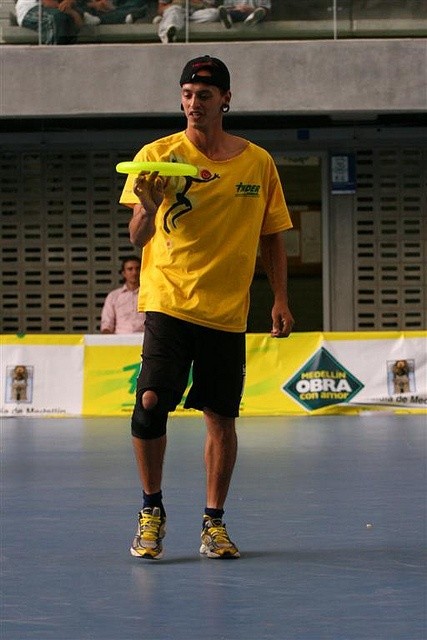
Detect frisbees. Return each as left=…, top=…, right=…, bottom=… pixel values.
left=116, top=162, right=198, bottom=176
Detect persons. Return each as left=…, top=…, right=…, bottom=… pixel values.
left=84, top=0, right=151, bottom=26
left=218, top=0, right=272, bottom=29
left=157, top=0, right=220, bottom=43
left=118, top=55, right=295, bottom=560
left=99, top=256, right=147, bottom=334
left=14, top=0, right=85, bottom=45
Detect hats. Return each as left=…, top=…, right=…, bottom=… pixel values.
left=179, top=55, right=229, bottom=92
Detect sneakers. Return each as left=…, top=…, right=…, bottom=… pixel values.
left=130, top=506, right=166, bottom=560
left=81, top=12, right=100, bottom=24
left=244, top=7, right=266, bottom=26
left=199, top=515, right=240, bottom=558
left=218, top=5, right=231, bottom=29
left=166, top=25, right=176, bottom=44
